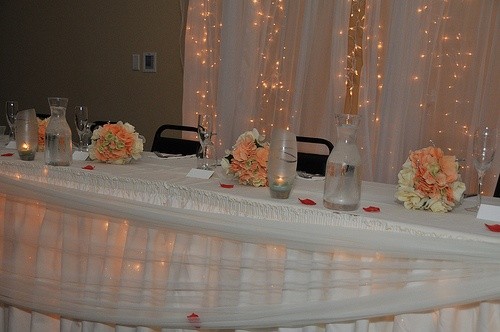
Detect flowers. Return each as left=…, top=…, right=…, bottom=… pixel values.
left=396, top=149, right=469, bottom=212
left=86, top=120, right=143, bottom=163
left=220, top=126, right=270, bottom=189
left=36, top=116, right=52, bottom=146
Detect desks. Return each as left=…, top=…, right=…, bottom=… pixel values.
left=0, top=134, right=500, bottom=332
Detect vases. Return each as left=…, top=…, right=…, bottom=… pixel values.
left=111, top=157, right=132, bottom=166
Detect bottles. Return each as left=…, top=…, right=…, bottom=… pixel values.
left=324, top=112, right=363, bottom=211
left=43, top=96, right=73, bottom=167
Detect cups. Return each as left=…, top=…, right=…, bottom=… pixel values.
left=266, top=127, right=298, bottom=200
left=81, top=122, right=96, bottom=151
left=14, top=108, right=38, bottom=161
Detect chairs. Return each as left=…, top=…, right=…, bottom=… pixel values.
left=296, top=136, right=333, bottom=176
left=89, top=120, right=116, bottom=143
left=152, top=124, right=210, bottom=158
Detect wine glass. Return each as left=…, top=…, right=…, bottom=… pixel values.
left=5, top=99, right=20, bottom=145
left=464, top=127, right=497, bottom=213
left=74, top=106, right=89, bottom=151
left=195, top=112, right=217, bottom=171
